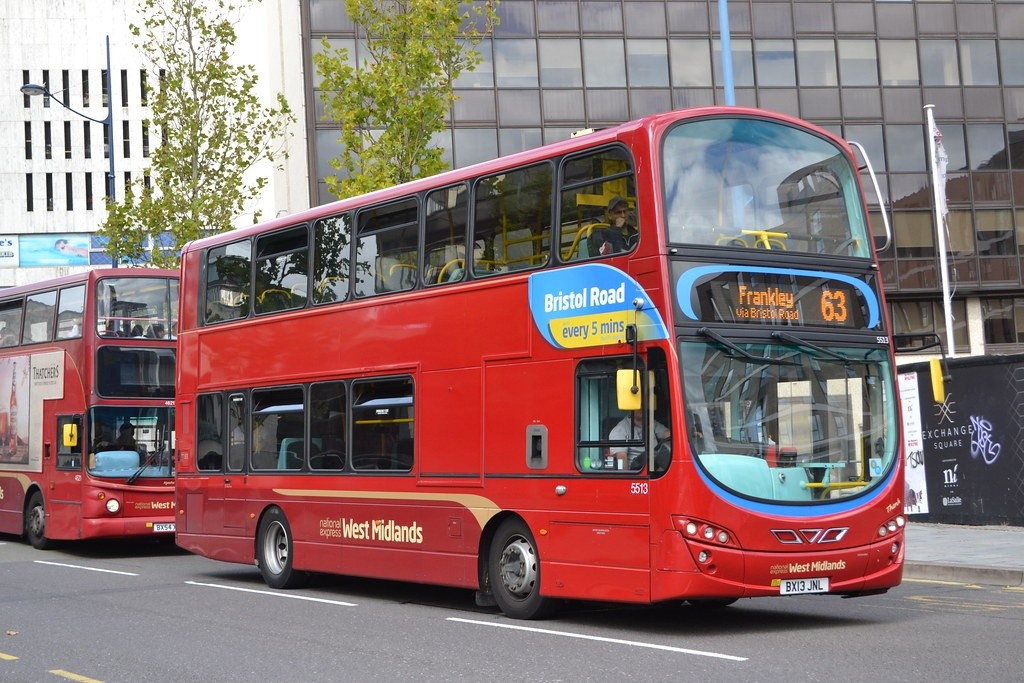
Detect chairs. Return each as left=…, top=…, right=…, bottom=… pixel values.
left=0, top=334, right=16, bottom=347
left=715, top=237, right=787, bottom=251
left=98, top=330, right=119, bottom=337
left=206, top=223, right=637, bottom=324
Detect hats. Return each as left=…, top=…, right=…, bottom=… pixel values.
left=120, top=423, right=135, bottom=432
left=608, top=198, right=630, bottom=213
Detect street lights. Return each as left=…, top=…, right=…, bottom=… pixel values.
left=18, top=34, right=121, bottom=267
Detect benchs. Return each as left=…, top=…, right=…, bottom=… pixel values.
left=277, top=437, right=343, bottom=470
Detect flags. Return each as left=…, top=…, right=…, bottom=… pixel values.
left=930, top=113, right=948, bottom=217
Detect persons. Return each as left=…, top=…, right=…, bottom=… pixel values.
left=609, top=410, right=670, bottom=472
left=131, top=324, right=171, bottom=339
left=104, top=423, right=146, bottom=458
left=592, top=197, right=638, bottom=255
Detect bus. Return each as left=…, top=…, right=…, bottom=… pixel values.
left=173, top=109, right=956, bottom=622
left=1, top=268, right=260, bottom=551
